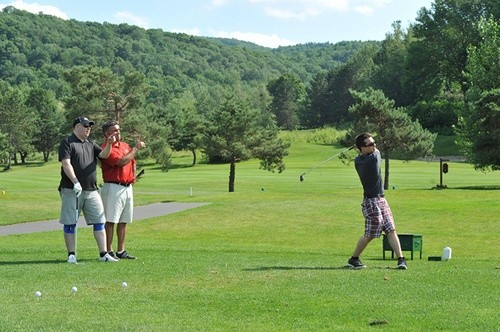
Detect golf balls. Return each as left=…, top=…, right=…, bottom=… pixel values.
left=71, top=287, right=77, bottom=293
left=122, top=282, right=127, bottom=288
left=35, top=291, right=41, bottom=297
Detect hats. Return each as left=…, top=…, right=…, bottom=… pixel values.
left=73, top=116, right=94, bottom=128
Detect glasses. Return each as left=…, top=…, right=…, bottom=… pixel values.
left=365, top=140, right=376, bottom=146
left=83, top=124, right=91, bottom=128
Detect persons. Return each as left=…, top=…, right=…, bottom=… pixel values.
left=56, top=116, right=119, bottom=262
left=347, top=133, right=407, bottom=269
left=98, top=121, right=145, bottom=262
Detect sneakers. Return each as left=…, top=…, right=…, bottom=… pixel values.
left=397, top=256, right=407, bottom=270
left=68, top=254, right=77, bottom=264
left=107, top=250, right=120, bottom=260
left=116, top=250, right=137, bottom=259
left=99, top=253, right=118, bottom=262
left=348, top=256, right=366, bottom=269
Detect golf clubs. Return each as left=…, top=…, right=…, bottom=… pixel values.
left=299, top=144, right=355, bottom=181
left=74, top=192, right=79, bottom=264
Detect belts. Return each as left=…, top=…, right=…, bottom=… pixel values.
left=364, top=193, right=385, bottom=198
left=104, top=180, right=130, bottom=186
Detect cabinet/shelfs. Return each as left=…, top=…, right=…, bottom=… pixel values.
left=381, top=234, right=423, bottom=260
